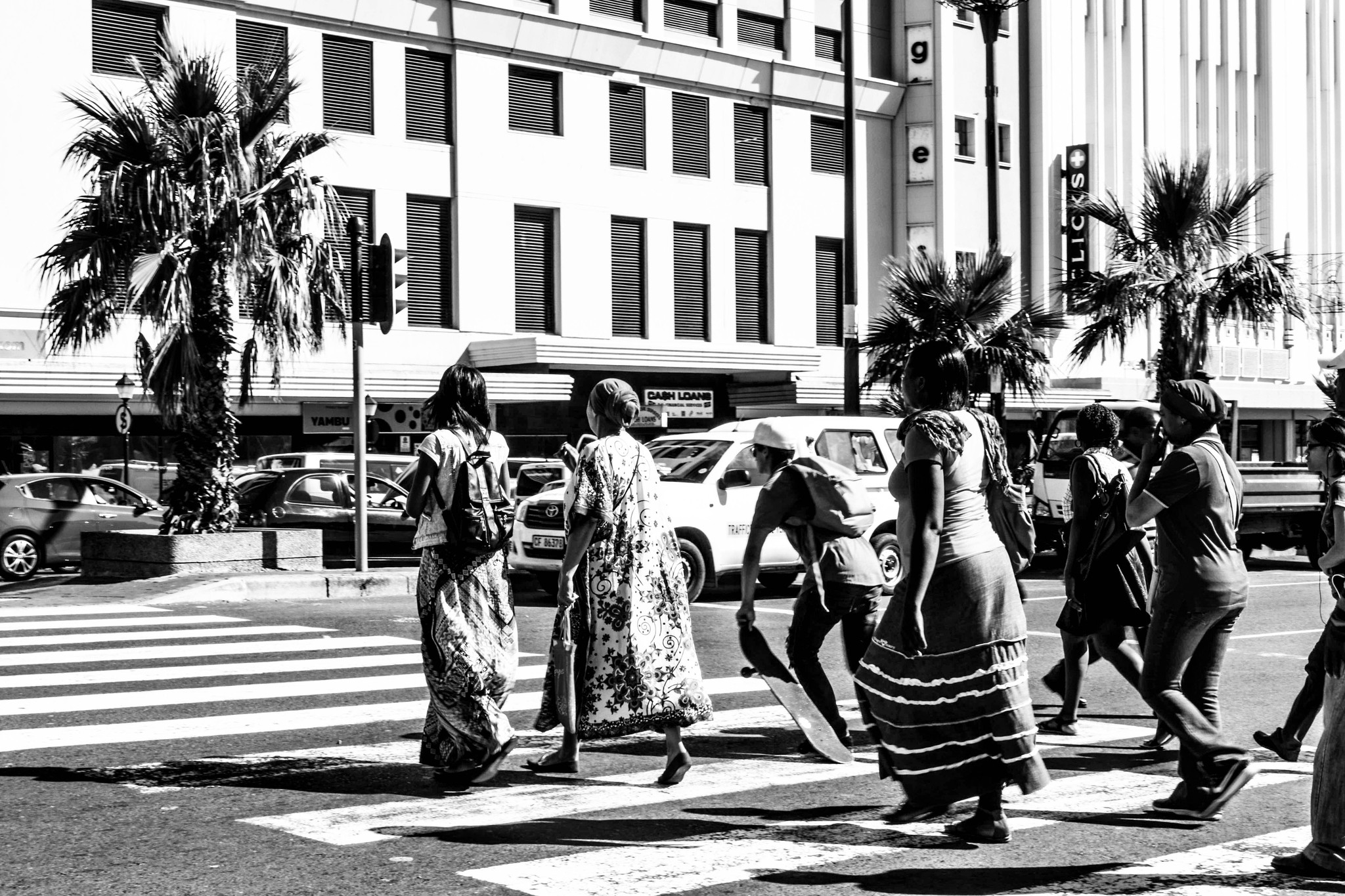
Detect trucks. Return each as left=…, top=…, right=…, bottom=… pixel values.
left=1033, top=400, right=1326, bottom=568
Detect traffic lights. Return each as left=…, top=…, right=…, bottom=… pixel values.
left=379, top=232, right=410, bottom=335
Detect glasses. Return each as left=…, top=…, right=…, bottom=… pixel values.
left=750, top=445, right=767, bottom=457
left=1307, top=442, right=1345, bottom=451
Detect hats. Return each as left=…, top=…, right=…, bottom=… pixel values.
left=751, top=420, right=797, bottom=450
left=591, top=378, right=641, bottom=428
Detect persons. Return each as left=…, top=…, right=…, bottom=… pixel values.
left=1037, top=405, right=1182, bottom=747
left=853, top=340, right=1052, bottom=844
left=1252, top=350, right=1345, bottom=761
left=525, top=378, right=713, bottom=786
left=735, top=421, right=888, bottom=746
left=404, top=364, right=519, bottom=783
left=1270, top=418, right=1345, bottom=880
left=1126, top=379, right=1263, bottom=817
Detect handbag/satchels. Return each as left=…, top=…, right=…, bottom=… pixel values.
left=969, top=409, right=1037, bottom=574
left=551, top=605, right=579, bottom=736
left=1058, top=454, right=1146, bottom=585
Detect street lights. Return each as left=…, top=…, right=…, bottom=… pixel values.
left=943, top=0, right=1018, bottom=472
left=116, top=373, right=135, bottom=501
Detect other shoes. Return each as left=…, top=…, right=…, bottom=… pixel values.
left=526, top=752, right=579, bottom=772
left=1253, top=727, right=1301, bottom=762
left=1139, top=725, right=1174, bottom=748
left=470, top=735, right=519, bottom=783
left=796, top=728, right=854, bottom=753
left=1199, top=758, right=1260, bottom=817
left=881, top=796, right=950, bottom=825
left=438, top=765, right=482, bottom=781
left=944, top=807, right=1012, bottom=842
left=1152, top=781, right=1225, bottom=820
left=1036, top=717, right=1079, bottom=735
left=1272, top=852, right=1341, bottom=878
left=1041, top=664, right=1088, bottom=708
left=658, top=751, right=692, bottom=784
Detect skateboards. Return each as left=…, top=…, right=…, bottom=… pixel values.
left=737, top=624, right=855, bottom=764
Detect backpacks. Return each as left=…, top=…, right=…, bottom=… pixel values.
left=431, top=427, right=516, bottom=552
left=776, top=454, right=876, bottom=536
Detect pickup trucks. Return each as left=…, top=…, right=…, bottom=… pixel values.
left=512, top=412, right=907, bottom=606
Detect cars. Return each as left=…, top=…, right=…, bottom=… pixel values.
left=0, top=473, right=199, bottom=582
left=229, top=466, right=427, bottom=564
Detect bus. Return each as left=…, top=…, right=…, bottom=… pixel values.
left=255, top=451, right=564, bottom=509
left=96, top=460, right=178, bottom=504
